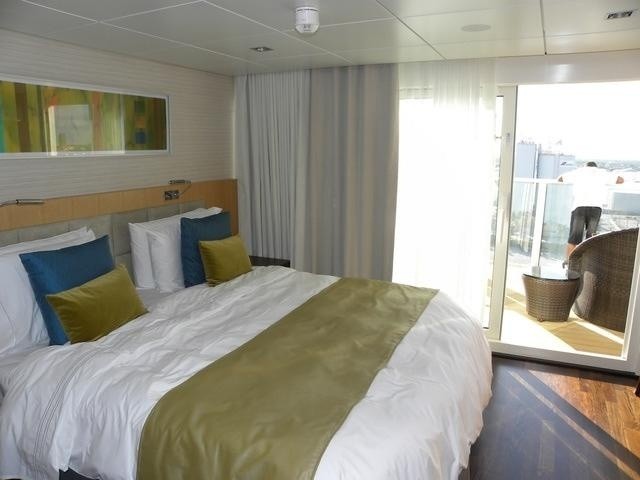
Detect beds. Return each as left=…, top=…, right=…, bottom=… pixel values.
left=0, top=179, right=492, bottom=479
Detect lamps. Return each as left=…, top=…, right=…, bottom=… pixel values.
left=294, top=6, right=320, bottom=35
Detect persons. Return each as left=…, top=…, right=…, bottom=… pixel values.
left=556, top=161, right=625, bottom=269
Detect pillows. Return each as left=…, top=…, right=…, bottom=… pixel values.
left=145, top=205, right=223, bottom=293
left=0, top=229, right=96, bottom=358
left=44, top=262, right=149, bottom=344
left=180, top=211, right=233, bottom=288
left=18, top=233, right=116, bottom=345
left=0, top=226, right=87, bottom=255
left=127, top=207, right=207, bottom=290
left=198, top=233, right=254, bottom=288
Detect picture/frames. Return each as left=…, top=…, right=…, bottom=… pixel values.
left=0, top=72, right=174, bottom=159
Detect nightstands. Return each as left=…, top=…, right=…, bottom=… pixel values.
left=249, top=254, right=290, bottom=268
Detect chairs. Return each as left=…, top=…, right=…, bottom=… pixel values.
left=567, top=226, right=640, bottom=332
